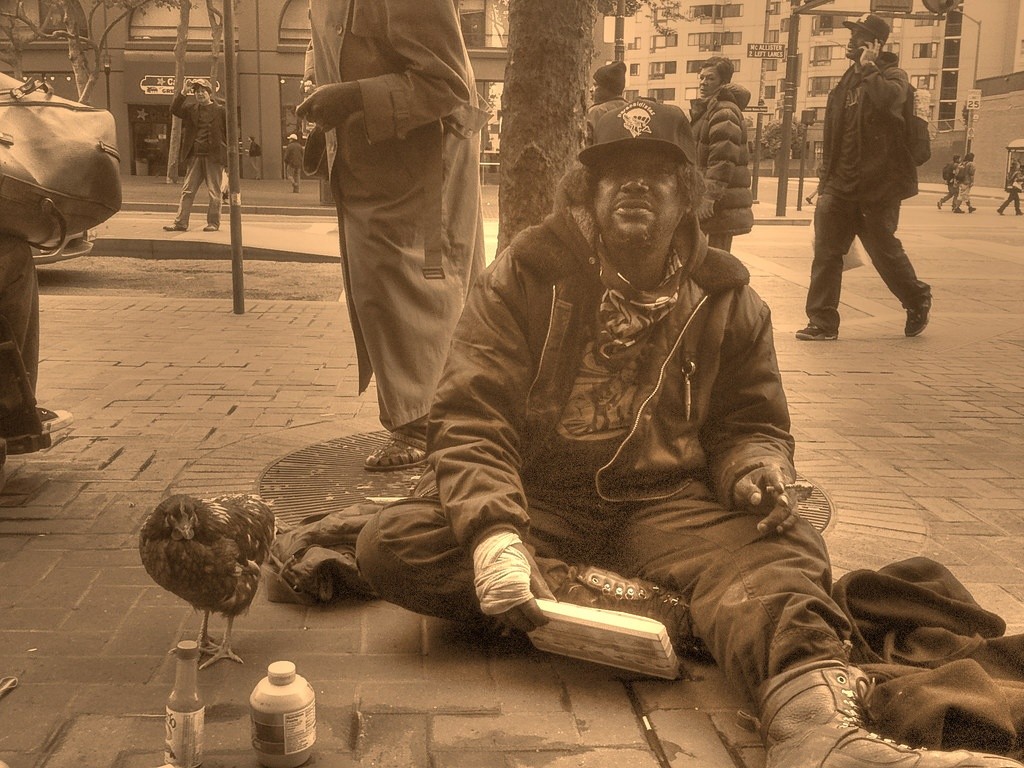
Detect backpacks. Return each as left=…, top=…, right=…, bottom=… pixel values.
left=955, top=161, right=968, bottom=181
left=882, top=67, right=931, bottom=167
left=943, top=163, right=955, bottom=180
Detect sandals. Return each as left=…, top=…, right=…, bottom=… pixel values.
left=363, top=429, right=431, bottom=471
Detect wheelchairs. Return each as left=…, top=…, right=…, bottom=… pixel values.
left=0, top=311, right=52, bottom=501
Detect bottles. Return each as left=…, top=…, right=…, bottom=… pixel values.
left=248, top=660, right=317, bottom=768
left=163, top=638, right=206, bottom=768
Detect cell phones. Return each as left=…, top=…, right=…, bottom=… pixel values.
left=859, top=41, right=886, bottom=55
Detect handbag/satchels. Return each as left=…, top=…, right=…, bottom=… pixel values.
left=219, top=170, right=241, bottom=209
left=0, top=72, right=123, bottom=248
left=811, top=211, right=862, bottom=272
left=1012, top=179, right=1023, bottom=192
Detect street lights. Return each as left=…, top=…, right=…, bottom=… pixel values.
left=103, top=55, right=112, bottom=112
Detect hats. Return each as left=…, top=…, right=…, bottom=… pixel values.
left=286, top=134, right=298, bottom=140
left=578, top=101, right=697, bottom=170
left=192, top=80, right=213, bottom=93
left=841, top=14, right=890, bottom=44
left=593, top=62, right=627, bottom=94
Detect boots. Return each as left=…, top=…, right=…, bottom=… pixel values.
left=558, top=562, right=715, bottom=662
left=737, top=654, right=1022, bottom=768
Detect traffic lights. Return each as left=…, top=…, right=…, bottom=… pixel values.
left=802, top=109, right=816, bottom=125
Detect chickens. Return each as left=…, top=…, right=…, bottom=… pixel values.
left=138, top=492, right=279, bottom=669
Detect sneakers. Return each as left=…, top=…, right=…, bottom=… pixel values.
left=796, top=323, right=837, bottom=341
left=904, top=296, right=932, bottom=337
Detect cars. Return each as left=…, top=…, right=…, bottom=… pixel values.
left=31, top=228, right=98, bottom=265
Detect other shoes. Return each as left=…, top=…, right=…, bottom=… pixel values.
left=954, top=208, right=965, bottom=213
left=969, top=206, right=976, bottom=213
left=1016, top=211, right=1023, bottom=216
left=938, top=201, right=942, bottom=210
left=163, top=224, right=185, bottom=231
left=997, top=208, right=1005, bottom=215
left=204, top=224, right=218, bottom=232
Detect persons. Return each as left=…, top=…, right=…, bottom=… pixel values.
left=795, top=13, right=933, bottom=341
left=291, top=0, right=488, bottom=468
left=0, top=231, right=77, bottom=436
left=937, top=152, right=1024, bottom=216
left=284, top=133, right=304, bottom=192
left=161, top=78, right=229, bottom=232
left=583, top=57, right=755, bottom=253
left=354, top=101, right=1024, bottom=768
left=246, top=137, right=262, bottom=181
